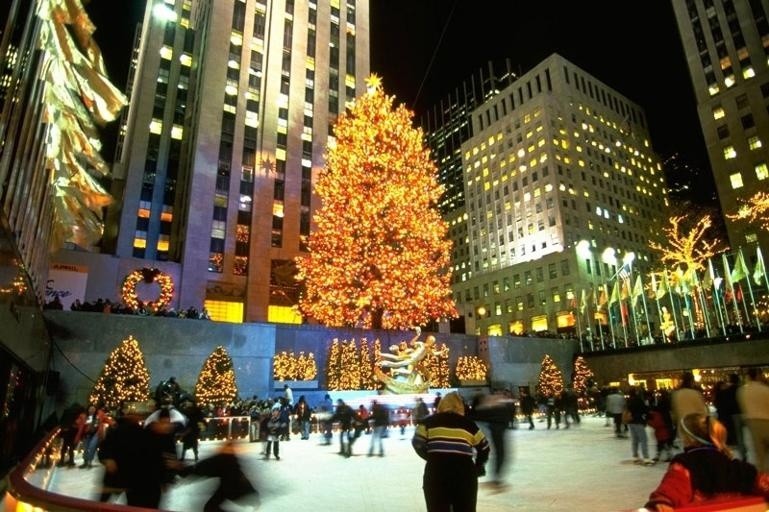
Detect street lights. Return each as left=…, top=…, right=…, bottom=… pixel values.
left=603, top=247, right=628, bottom=349
left=576, top=239, right=605, bottom=351
left=622, top=251, right=641, bottom=348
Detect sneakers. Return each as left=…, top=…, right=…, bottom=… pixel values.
left=59, top=460, right=92, bottom=469
left=632, top=456, right=674, bottom=464
left=301, top=436, right=309, bottom=440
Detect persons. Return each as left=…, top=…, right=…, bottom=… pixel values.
left=412, top=393, right=490, bottom=511
left=46, top=296, right=209, bottom=320
left=639, top=413, right=769, bottom=510
left=97, top=407, right=257, bottom=512
left=507, top=324, right=769, bottom=351
left=52, top=368, right=769, bottom=474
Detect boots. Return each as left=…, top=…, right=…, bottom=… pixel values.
left=338, top=444, right=386, bottom=458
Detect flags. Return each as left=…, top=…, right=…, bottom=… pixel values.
left=35, top=0, right=129, bottom=252
left=579, top=249, right=764, bottom=315
